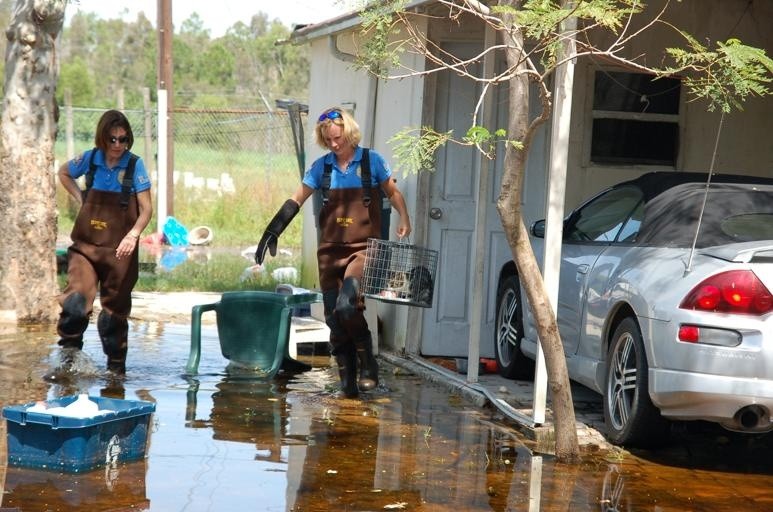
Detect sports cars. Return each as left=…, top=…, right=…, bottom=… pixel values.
left=494, top=100, right=773, bottom=449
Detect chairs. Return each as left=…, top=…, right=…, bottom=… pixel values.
left=183, top=290, right=316, bottom=377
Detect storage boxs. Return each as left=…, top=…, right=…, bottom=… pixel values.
left=0, top=394, right=155, bottom=474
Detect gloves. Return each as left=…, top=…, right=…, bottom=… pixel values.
left=253, top=198, right=299, bottom=266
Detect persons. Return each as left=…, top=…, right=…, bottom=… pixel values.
left=41, top=109, right=153, bottom=389
left=254, top=105, right=412, bottom=401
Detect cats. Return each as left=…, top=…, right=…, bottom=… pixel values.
left=389, top=265, right=433, bottom=305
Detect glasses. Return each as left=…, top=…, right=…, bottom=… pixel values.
left=108, top=135, right=130, bottom=144
left=318, top=111, right=340, bottom=121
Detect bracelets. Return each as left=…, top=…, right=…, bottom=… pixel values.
left=128, top=232, right=138, bottom=239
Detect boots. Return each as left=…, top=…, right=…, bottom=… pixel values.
left=354, top=330, right=381, bottom=393
left=330, top=348, right=360, bottom=400
left=100, top=357, right=126, bottom=396
left=44, top=339, right=80, bottom=384
